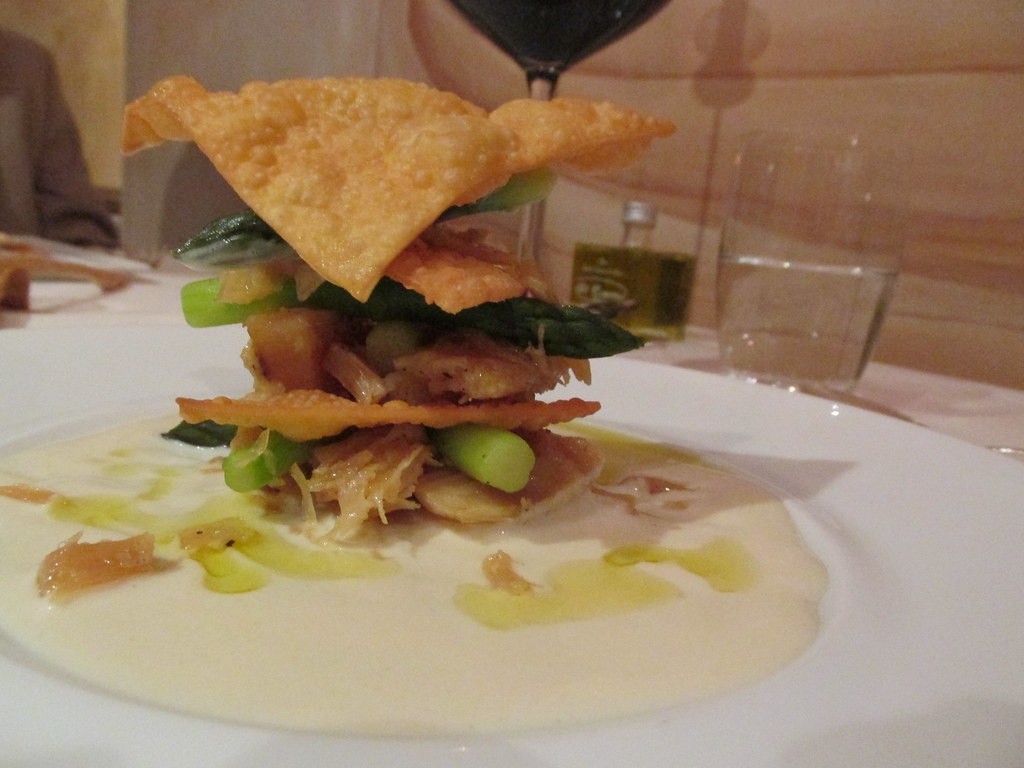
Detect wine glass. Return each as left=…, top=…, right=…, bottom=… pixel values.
left=441, top=0, right=676, bottom=300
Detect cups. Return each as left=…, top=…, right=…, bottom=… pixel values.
left=714, top=128, right=915, bottom=404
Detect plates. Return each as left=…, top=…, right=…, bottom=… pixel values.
left=0, top=312, right=1024, bottom=768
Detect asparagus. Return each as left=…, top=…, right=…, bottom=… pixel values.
left=158, top=400, right=536, bottom=494
left=167, top=162, right=558, bottom=269
left=181, top=272, right=651, bottom=359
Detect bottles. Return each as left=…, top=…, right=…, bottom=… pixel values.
left=568, top=198, right=699, bottom=344
